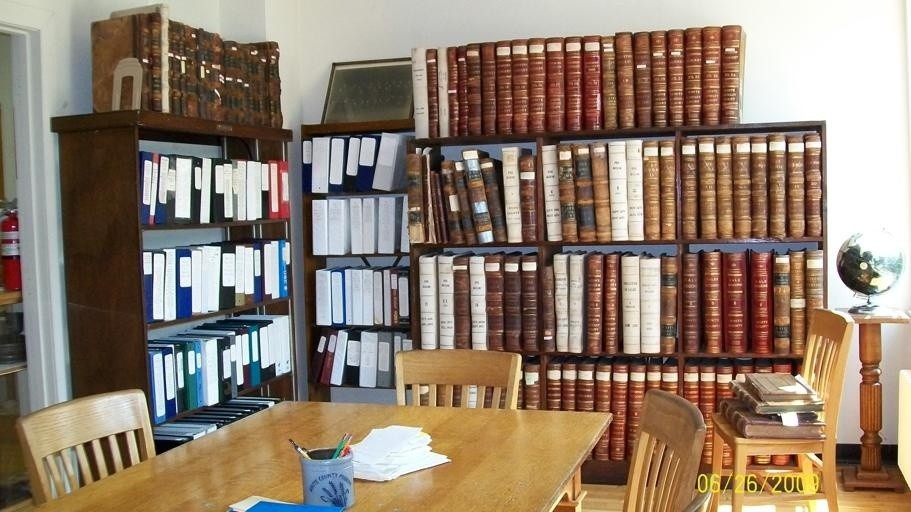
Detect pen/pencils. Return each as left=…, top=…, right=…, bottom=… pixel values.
left=288, top=432, right=353, bottom=460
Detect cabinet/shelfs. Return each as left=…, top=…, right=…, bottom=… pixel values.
left=302, top=119, right=415, bottom=403
left=0, top=291, right=26, bottom=376
left=409, top=120, right=825, bottom=490
left=49, top=111, right=296, bottom=491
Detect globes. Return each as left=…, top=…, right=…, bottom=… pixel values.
left=837, top=227, right=903, bottom=314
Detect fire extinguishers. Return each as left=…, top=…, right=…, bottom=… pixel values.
left=0, top=208, right=22, bottom=291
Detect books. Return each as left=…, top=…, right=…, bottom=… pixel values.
left=90, top=6, right=283, bottom=127
left=407, top=24, right=825, bottom=470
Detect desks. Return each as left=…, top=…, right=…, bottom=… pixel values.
left=16, top=402, right=613, bottom=512
left=835, top=307, right=910, bottom=492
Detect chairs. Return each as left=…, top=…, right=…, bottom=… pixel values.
left=710, top=307, right=854, bottom=512
left=622, top=387, right=706, bottom=512
left=683, top=475, right=715, bottom=512
left=394, top=349, right=521, bottom=411
left=17, top=388, right=157, bottom=505
